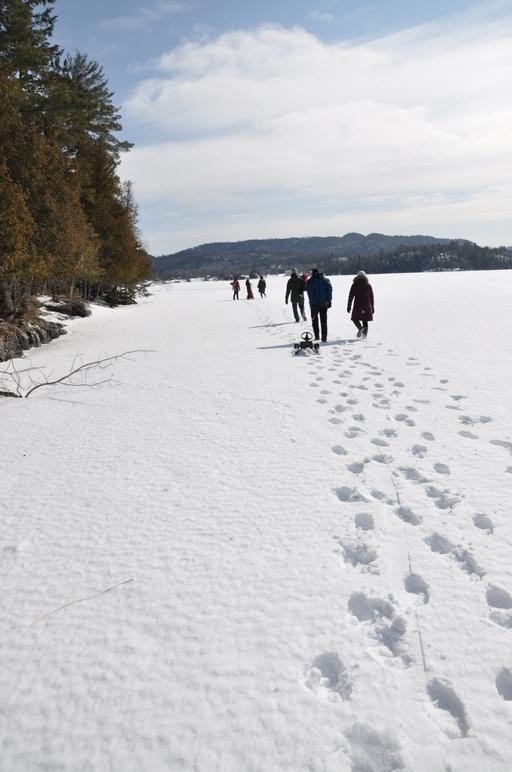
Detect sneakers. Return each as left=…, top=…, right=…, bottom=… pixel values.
left=356, top=328, right=367, bottom=340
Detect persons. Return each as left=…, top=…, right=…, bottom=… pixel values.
left=307, top=269, right=332, bottom=342
left=258, top=276, right=266, bottom=298
left=246, top=278, right=254, bottom=299
left=347, top=270, right=374, bottom=337
left=285, top=267, right=308, bottom=322
left=230, top=277, right=240, bottom=300
